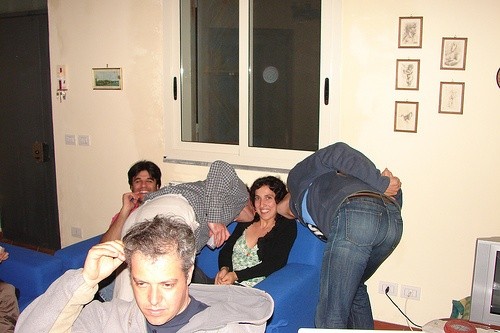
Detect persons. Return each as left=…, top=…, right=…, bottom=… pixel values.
left=14, top=215, right=275, bottom=333
left=0, top=280, right=19, bottom=333
left=214, top=175, right=297, bottom=287
left=276, top=143, right=403, bottom=329
left=100, top=160, right=161, bottom=246
left=113, top=161, right=255, bottom=304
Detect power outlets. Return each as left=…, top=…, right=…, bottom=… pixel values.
left=400, top=284, right=422, bottom=302
left=377, top=280, right=398, bottom=297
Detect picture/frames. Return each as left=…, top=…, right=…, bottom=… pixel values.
left=394, top=100, right=419, bottom=134
left=398, top=16, right=423, bottom=49
left=438, top=81, right=466, bottom=115
left=395, top=58, right=421, bottom=90
left=440, top=37, right=467, bottom=71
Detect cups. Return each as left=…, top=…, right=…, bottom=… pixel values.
left=206, top=234, right=217, bottom=250
left=444, top=319, right=477, bottom=333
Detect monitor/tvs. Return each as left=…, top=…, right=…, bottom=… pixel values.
left=470, top=236, right=500, bottom=328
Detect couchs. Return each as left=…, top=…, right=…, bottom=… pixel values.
left=0, top=241, right=61, bottom=314
left=58, top=219, right=329, bottom=333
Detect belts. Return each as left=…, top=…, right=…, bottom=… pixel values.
left=347, top=191, right=394, bottom=204
